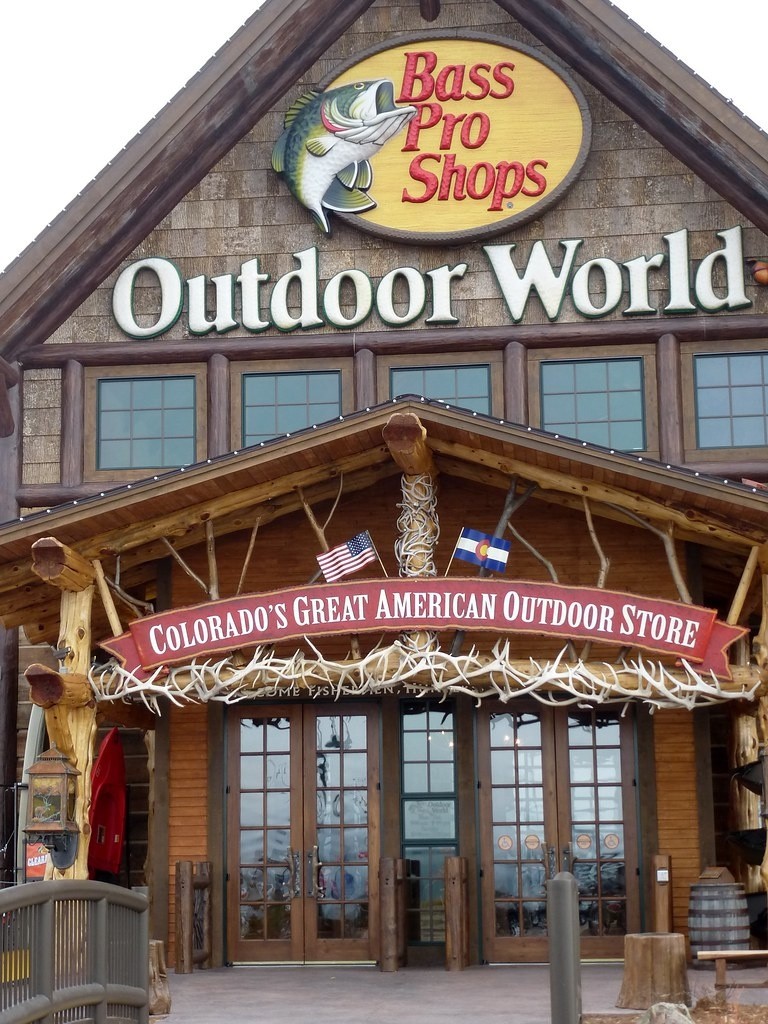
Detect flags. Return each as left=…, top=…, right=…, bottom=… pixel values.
left=317, top=532, right=378, bottom=582
left=454, top=528, right=511, bottom=574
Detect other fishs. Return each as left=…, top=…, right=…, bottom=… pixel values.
left=271, top=79, right=418, bottom=233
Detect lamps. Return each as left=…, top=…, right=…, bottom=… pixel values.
left=21, top=741, right=83, bottom=854
